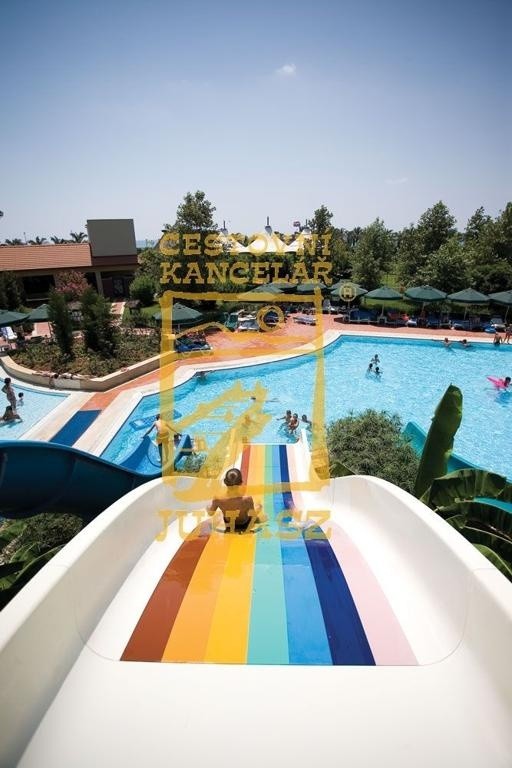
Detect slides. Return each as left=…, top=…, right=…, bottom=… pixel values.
left=1, top=429, right=512, bottom=768
left=2, top=434, right=192, bottom=522
left=330, top=419, right=512, bottom=516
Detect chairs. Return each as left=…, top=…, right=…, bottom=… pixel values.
left=225, top=314, right=238, bottom=330
left=293, top=316, right=317, bottom=325
left=348, top=309, right=507, bottom=333
left=1, top=326, right=18, bottom=350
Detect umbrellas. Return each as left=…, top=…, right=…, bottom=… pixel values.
left=27, top=303, right=60, bottom=337
left=153, top=302, right=205, bottom=332
left=245, top=276, right=511, bottom=326
left=0, top=308, right=31, bottom=348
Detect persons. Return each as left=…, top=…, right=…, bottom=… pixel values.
left=2, top=377, right=18, bottom=409
left=374, top=366, right=383, bottom=377
left=173, top=435, right=181, bottom=445
left=139, top=412, right=180, bottom=463
left=32, top=369, right=67, bottom=379
left=285, top=413, right=300, bottom=438
left=459, top=338, right=473, bottom=347
left=365, top=363, right=374, bottom=377
left=17, top=391, right=25, bottom=406
left=277, top=410, right=291, bottom=435
left=493, top=331, right=501, bottom=345
left=503, top=323, right=512, bottom=344
left=298, top=414, right=312, bottom=424
left=370, top=353, right=380, bottom=365
left=1, top=406, right=23, bottom=424
left=486, top=374, right=511, bottom=393
left=442, top=336, right=451, bottom=347
left=250, top=395, right=280, bottom=403
left=204, top=467, right=273, bottom=533
left=15, top=331, right=26, bottom=346
left=49, top=373, right=59, bottom=389
